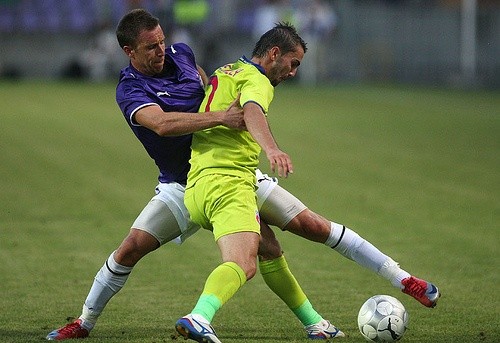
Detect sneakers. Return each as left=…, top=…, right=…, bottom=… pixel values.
left=174, top=314, right=221, bottom=343
left=45, top=318, right=89, bottom=341
left=305, top=319, right=344, bottom=339
left=402, top=275, right=440, bottom=308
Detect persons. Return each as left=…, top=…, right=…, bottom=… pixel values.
left=175, top=21, right=348, bottom=341
left=19, top=7, right=441, bottom=342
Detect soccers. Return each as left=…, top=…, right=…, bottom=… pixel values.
left=356, top=295, right=409, bottom=343
